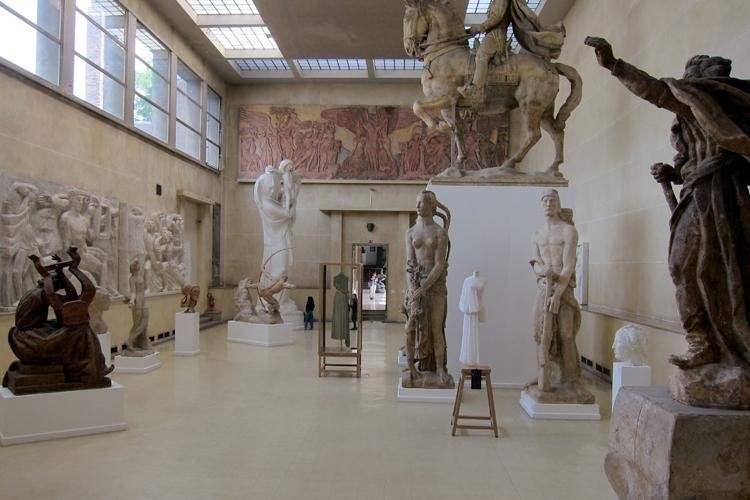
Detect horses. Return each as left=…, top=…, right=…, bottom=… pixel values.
left=401, top=0, right=583, bottom=175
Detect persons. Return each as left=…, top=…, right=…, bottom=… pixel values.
left=305, top=295, right=315, bottom=328
left=332, top=271, right=351, bottom=351
left=3, top=183, right=185, bottom=299
left=125, top=258, right=149, bottom=351
left=455, top=0, right=564, bottom=96
left=584, top=35, right=749, bottom=368
left=350, top=293, right=358, bottom=331
left=278, top=159, right=298, bottom=210
left=253, top=173, right=295, bottom=300
left=404, top=190, right=450, bottom=378
left=527, top=187, right=580, bottom=389
left=611, top=324, right=649, bottom=366
left=368, top=272, right=377, bottom=299
left=183, top=285, right=200, bottom=311
left=461, top=270, right=488, bottom=366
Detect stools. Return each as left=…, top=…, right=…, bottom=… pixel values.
left=452, top=368, right=499, bottom=438
